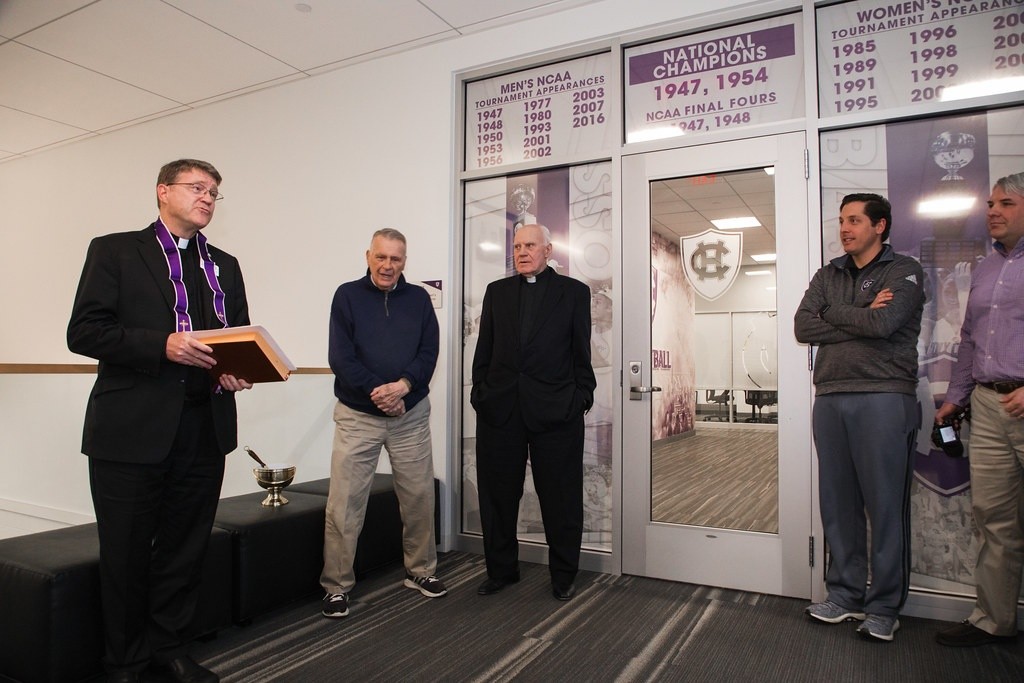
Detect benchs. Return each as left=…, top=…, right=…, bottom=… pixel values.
left=0, top=474, right=441, bottom=683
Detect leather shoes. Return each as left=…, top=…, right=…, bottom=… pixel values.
left=103, top=654, right=220, bottom=683
left=551, top=576, right=576, bottom=601
left=477, top=573, right=520, bottom=595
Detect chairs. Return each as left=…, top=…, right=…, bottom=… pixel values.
left=703, top=390, right=737, bottom=422
left=745, top=391, right=777, bottom=424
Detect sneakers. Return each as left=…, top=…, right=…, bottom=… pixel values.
left=404, top=571, right=447, bottom=598
left=321, top=591, right=350, bottom=617
left=856, top=614, right=900, bottom=641
left=805, top=600, right=866, bottom=624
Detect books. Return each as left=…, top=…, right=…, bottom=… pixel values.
left=191, top=330, right=291, bottom=383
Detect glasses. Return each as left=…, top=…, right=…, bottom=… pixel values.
left=166, top=182, right=223, bottom=200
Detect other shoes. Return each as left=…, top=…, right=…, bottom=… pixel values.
left=935, top=618, right=1018, bottom=647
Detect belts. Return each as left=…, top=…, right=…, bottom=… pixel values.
left=979, top=381, right=1024, bottom=394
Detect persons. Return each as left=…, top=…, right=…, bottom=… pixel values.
left=318, top=228, right=446, bottom=617
left=66, top=159, right=251, bottom=683
left=794, top=193, right=925, bottom=641
left=934, top=172, right=1024, bottom=647
left=469, top=224, right=598, bottom=601
